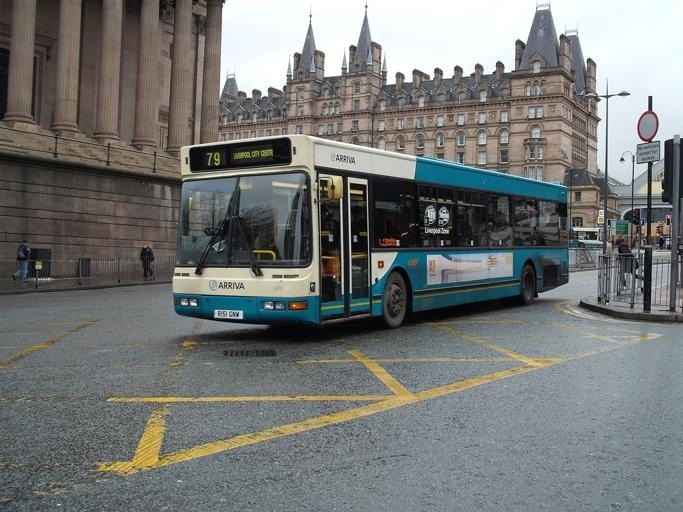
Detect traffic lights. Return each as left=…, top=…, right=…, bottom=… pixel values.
left=666, top=215, right=671, bottom=225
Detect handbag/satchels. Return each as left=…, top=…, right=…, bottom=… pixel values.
left=18, top=246, right=25, bottom=258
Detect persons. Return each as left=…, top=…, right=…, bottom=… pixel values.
left=138, top=240, right=156, bottom=282
left=630, top=236, right=637, bottom=249
left=13, top=240, right=31, bottom=282
left=616, top=236, right=643, bottom=289
left=658, top=236, right=666, bottom=250
left=607, top=240, right=612, bottom=254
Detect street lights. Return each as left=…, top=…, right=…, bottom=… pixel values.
left=585, top=79, right=636, bottom=303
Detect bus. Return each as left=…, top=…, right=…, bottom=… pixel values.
left=173, top=134, right=567, bottom=330
left=570, top=227, right=608, bottom=248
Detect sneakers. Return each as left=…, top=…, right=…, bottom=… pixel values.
left=12, top=273, right=16, bottom=280
left=23, top=280, right=27, bottom=283
